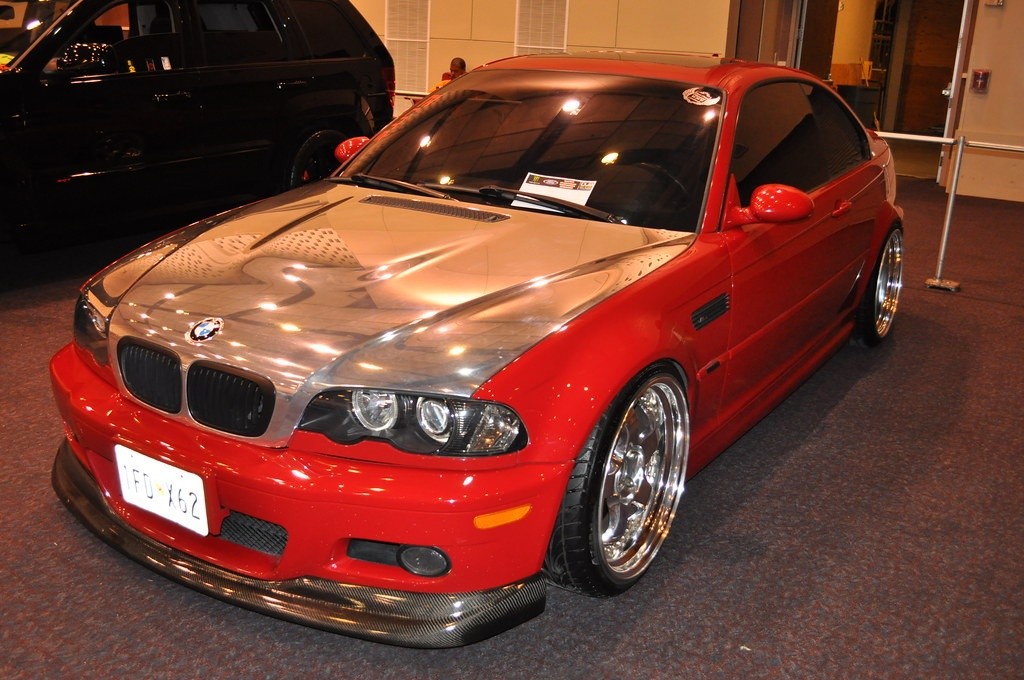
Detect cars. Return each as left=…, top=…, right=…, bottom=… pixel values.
left=0, top=0, right=395, bottom=254
left=51, top=51, right=905, bottom=648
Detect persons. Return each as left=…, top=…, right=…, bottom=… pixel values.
left=428, top=57, right=466, bottom=93
